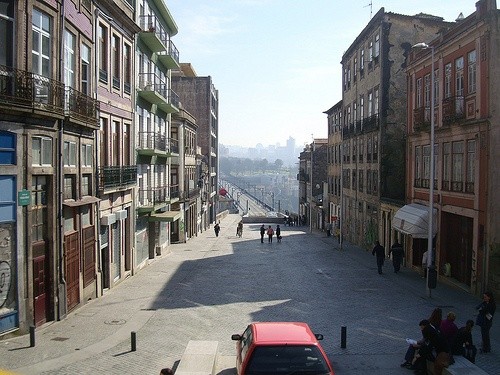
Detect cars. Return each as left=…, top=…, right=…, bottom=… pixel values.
left=230, top=321, right=335, bottom=375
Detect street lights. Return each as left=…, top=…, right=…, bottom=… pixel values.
left=412, top=41, right=436, bottom=298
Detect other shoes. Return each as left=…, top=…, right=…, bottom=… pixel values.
left=402, top=361, right=412, bottom=367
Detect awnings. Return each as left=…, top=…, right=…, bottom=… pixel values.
left=392, top=203, right=437, bottom=238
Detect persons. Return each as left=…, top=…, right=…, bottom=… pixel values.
left=452, top=319, right=477, bottom=364
left=427, top=351, right=452, bottom=375
left=284, top=215, right=307, bottom=227
left=238, top=220, right=243, bottom=238
left=400, top=319, right=441, bottom=370
left=214, top=223, right=220, bottom=237
left=411, top=325, right=456, bottom=375
left=260, top=225, right=267, bottom=243
left=436, top=312, right=458, bottom=337
left=267, top=225, right=274, bottom=244
left=372, top=241, right=385, bottom=274
left=422, top=250, right=435, bottom=281
left=476, top=291, right=496, bottom=354
left=276, top=225, right=281, bottom=243
left=325, top=221, right=331, bottom=237
left=387, top=238, right=405, bottom=273
